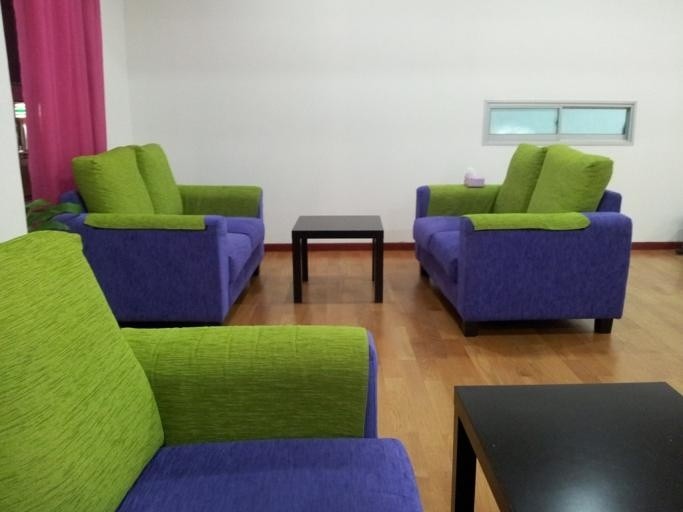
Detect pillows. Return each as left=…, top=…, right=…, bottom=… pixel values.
left=70, top=146, right=155, bottom=215
left=132, top=143, right=184, bottom=215
left=0, top=230, right=165, bottom=512
left=492, top=144, right=547, bottom=212
left=527, top=144, right=613, bottom=216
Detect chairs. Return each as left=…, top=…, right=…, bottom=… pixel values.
left=0, top=229, right=425, bottom=512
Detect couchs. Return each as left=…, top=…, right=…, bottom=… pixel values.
left=414, top=144, right=632, bottom=338
left=46, top=142, right=266, bottom=324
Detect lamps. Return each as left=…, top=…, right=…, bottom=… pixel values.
left=14, top=103, right=30, bottom=166
